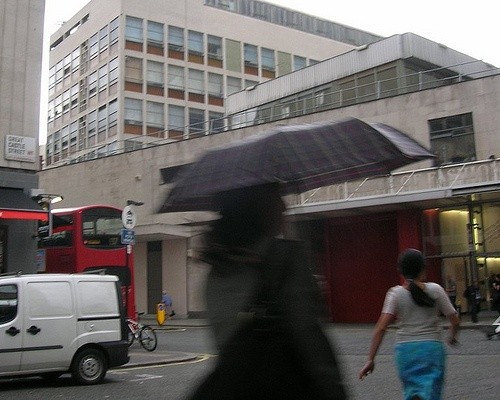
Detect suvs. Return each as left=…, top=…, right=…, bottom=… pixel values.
left=0, top=268, right=131, bottom=386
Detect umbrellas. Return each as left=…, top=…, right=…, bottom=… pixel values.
left=154, top=115, right=440, bottom=214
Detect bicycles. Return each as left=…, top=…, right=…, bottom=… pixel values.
left=124, top=310, right=158, bottom=352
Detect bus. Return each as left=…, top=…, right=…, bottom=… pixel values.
left=36, top=204, right=139, bottom=332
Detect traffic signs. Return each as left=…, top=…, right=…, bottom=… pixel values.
left=122, top=230, right=135, bottom=245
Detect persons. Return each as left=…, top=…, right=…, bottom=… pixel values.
left=187, top=186, right=345, bottom=400
left=444, top=275, right=461, bottom=315
left=357, top=247, right=461, bottom=400
left=463, top=279, right=482, bottom=325
left=161, top=289, right=176, bottom=320
left=492, top=274, right=500, bottom=315
